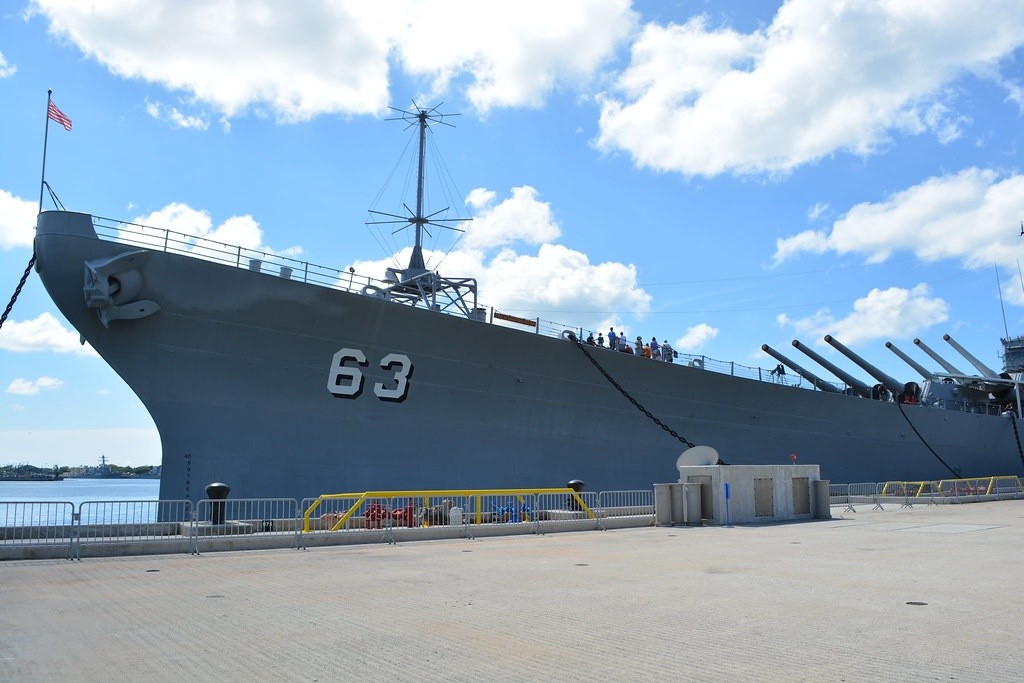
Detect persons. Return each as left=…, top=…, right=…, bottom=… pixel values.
left=588, top=327, right=674, bottom=365
left=1004, top=402, right=1014, bottom=412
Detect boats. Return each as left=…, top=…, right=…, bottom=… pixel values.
left=74, top=455, right=122, bottom=479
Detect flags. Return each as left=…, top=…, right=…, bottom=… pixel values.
left=45, top=100, right=71, bottom=135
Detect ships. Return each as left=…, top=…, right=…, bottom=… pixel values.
left=33, top=88, right=1024, bottom=522
